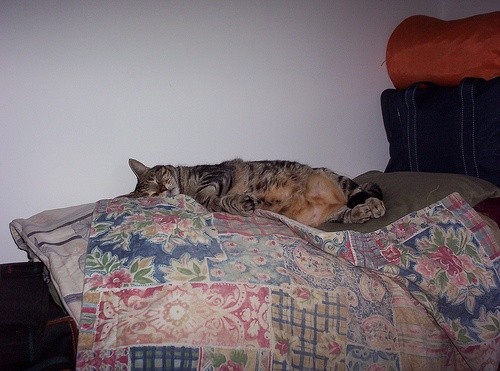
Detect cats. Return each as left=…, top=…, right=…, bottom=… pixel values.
left=128, top=157, right=386, bottom=228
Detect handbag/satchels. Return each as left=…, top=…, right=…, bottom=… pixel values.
left=385, top=10, right=500, bottom=89
left=380, top=77, right=500, bottom=188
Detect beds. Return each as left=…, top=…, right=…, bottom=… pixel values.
left=0, top=77, right=500, bottom=371
